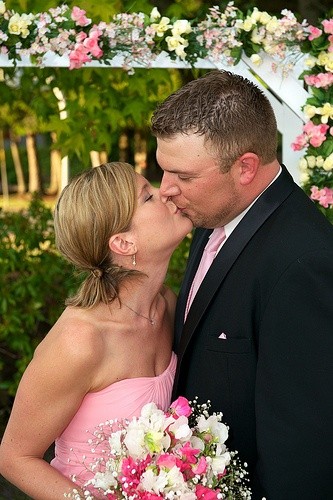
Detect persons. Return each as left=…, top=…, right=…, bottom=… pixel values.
left=0, top=162, right=193, bottom=500
left=151, top=69, right=333, bottom=500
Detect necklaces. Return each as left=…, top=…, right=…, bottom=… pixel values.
left=116, top=294, right=161, bottom=326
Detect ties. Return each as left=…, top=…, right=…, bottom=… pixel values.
left=184, top=227, right=226, bottom=323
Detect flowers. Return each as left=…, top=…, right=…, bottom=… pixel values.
left=58, top=396, right=253, bottom=500
left=0, top=2, right=333, bottom=223
